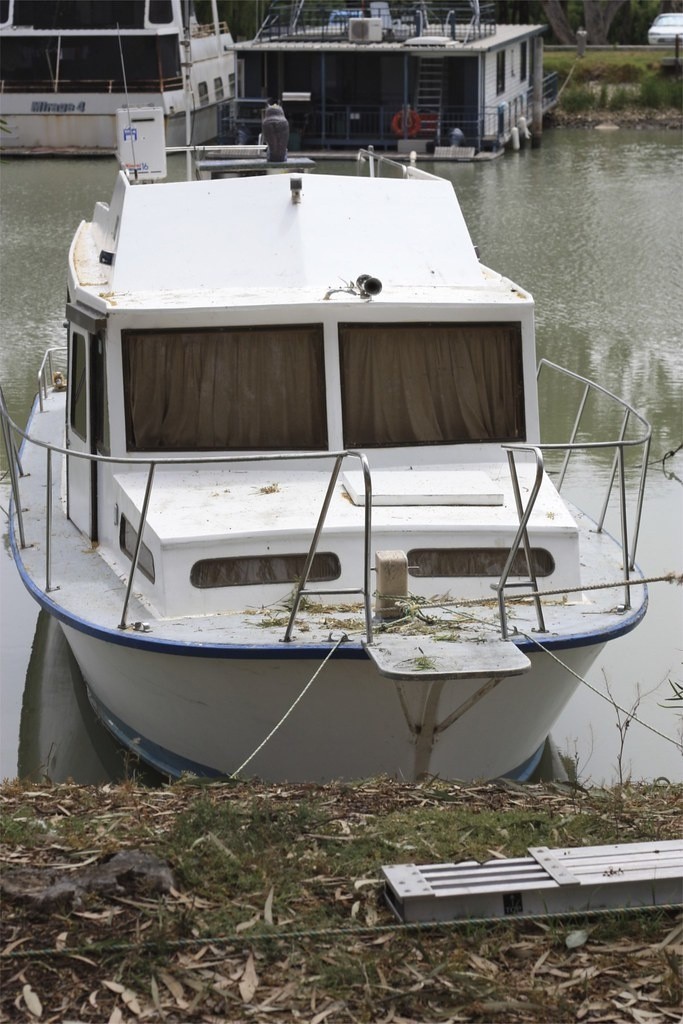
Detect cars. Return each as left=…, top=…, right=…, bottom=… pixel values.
left=648, top=13, right=683, bottom=45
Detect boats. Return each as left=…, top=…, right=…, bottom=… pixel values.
left=0, top=0, right=235, bottom=156
left=0, top=23, right=650, bottom=781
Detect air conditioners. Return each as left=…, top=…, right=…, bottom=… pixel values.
left=349, top=17, right=382, bottom=41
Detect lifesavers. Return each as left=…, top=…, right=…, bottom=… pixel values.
left=391, top=109, right=421, bottom=137
